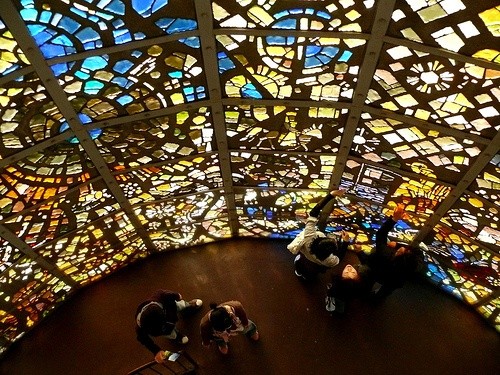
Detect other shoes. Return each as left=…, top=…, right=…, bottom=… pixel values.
left=175, top=332, right=188, bottom=344
left=218, top=344, right=228, bottom=354
left=251, top=331, right=259, bottom=341
left=188, top=299, right=202, bottom=309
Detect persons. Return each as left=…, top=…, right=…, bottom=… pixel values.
left=199, top=300, right=259, bottom=356
left=327, top=244, right=373, bottom=306
left=134, top=289, right=202, bottom=363
left=293, top=188, right=349, bottom=281
left=373, top=208, right=425, bottom=308
